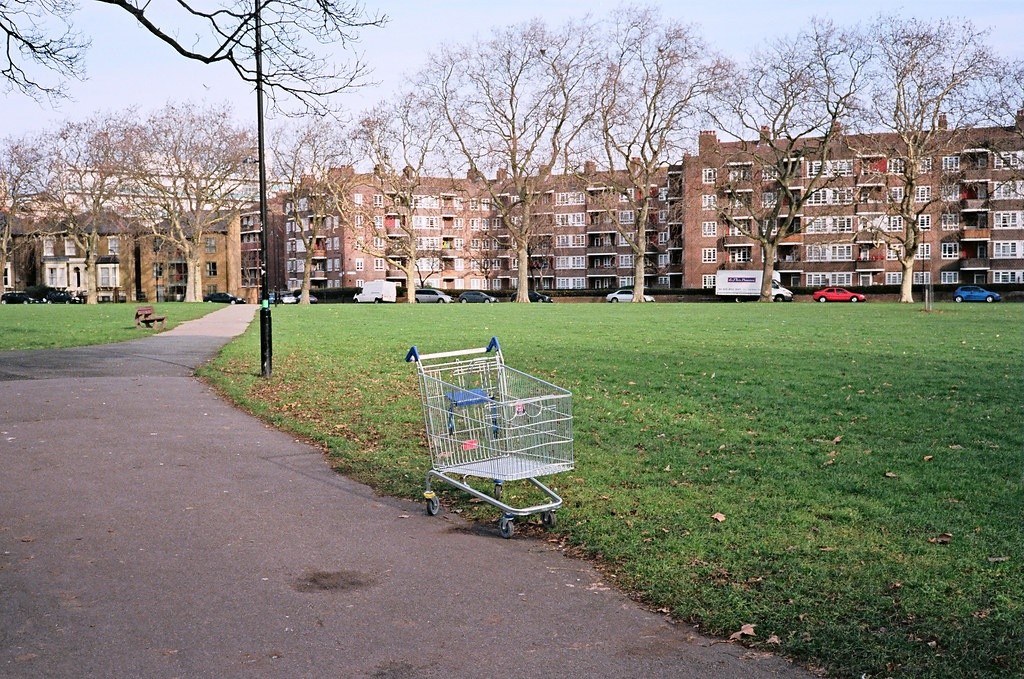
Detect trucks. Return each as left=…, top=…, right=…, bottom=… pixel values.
left=352, top=281, right=397, bottom=303
left=714, top=268, right=794, bottom=302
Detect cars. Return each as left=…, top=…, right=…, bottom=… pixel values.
left=1, top=293, right=40, bottom=304
left=812, top=288, right=867, bottom=303
left=458, top=291, right=500, bottom=303
left=203, top=293, right=247, bottom=304
left=269, top=293, right=279, bottom=303
left=42, top=291, right=80, bottom=304
left=953, top=286, right=1002, bottom=303
left=606, top=290, right=656, bottom=302
left=298, top=294, right=318, bottom=304
left=280, top=293, right=297, bottom=304
left=511, top=291, right=553, bottom=303
left=414, top=289, right=455, bottom=303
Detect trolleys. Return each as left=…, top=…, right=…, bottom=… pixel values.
left=406, top=336, right=575, bottom=540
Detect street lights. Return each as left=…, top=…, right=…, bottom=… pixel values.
left=922, top=227, right=931, bottom=301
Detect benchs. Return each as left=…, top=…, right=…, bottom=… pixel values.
left=136, top=305, right=166, bottom=332
